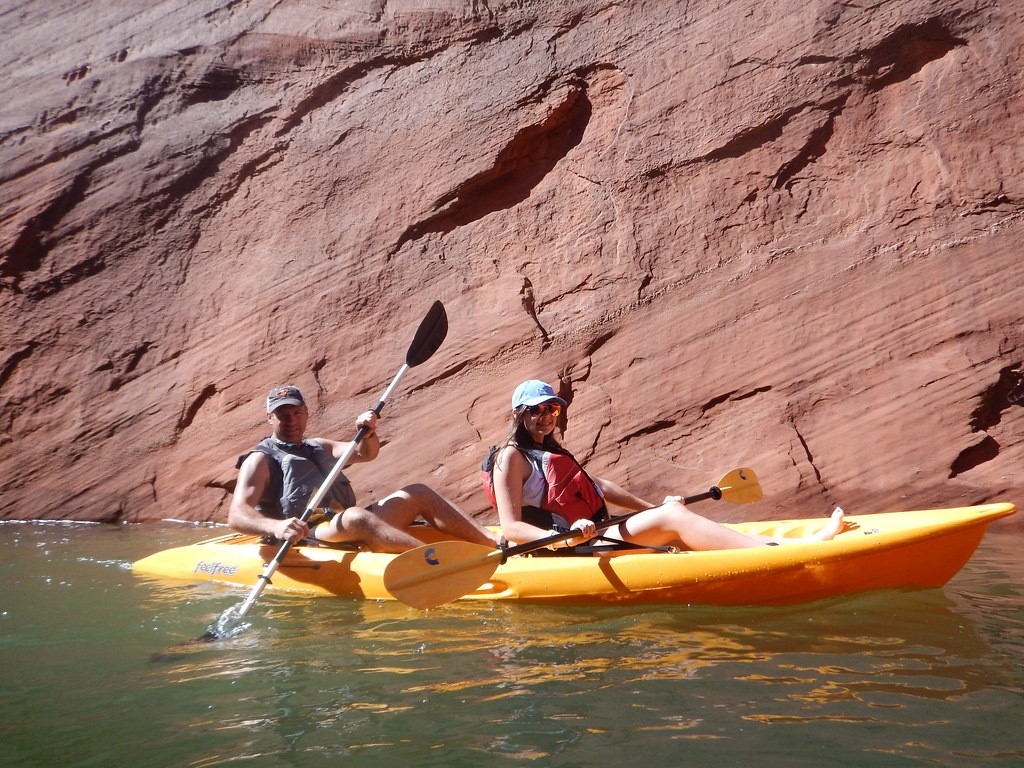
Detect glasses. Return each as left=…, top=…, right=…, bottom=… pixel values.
left=526, top=400, right=562, bottom=417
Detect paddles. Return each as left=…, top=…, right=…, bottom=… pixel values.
left=383, top=467, right=763, bottom=612
left=238, top=300, right=451, bottom=617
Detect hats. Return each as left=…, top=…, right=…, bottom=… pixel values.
left=511, top=380, right=567, bottom=409
left=267, top=386, right=303, bottom=414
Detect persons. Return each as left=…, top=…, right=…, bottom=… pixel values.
left=227, top=385, right=518, bottom=555
left=493, top=378, right=845, bottom=557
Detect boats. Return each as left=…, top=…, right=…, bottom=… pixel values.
left=133, top=495, right=1021, bottom=606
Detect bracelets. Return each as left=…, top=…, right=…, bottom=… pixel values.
left=565, top=539, right=573, bottom=549
left=546, top=529, right=559, bottom=552
